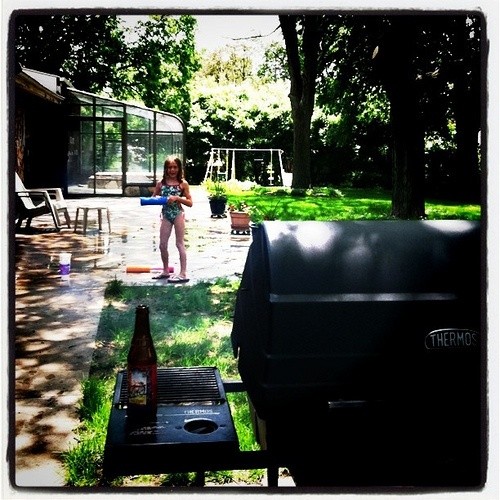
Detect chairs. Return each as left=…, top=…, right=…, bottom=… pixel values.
left=15, top=171, right=71, bottom=232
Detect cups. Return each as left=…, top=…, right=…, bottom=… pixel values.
left=58, top=253, right=72, bottom=280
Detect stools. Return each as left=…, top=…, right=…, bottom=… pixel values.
left=74, top=206, right=111, bottom=235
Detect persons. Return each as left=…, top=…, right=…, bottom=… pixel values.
left=151, top=155, right=194, bottom=283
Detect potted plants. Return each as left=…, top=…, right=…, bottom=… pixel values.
left=227, top=200, right=257, bottom=235
left=208, top=183, right=228, bottom=219
left=249, top=201, right=280, bottom=241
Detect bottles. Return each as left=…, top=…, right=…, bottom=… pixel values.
left=127, top=305, right=157, bottom=422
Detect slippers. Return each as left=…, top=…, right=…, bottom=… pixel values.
left=168, top=275, right=189, bottom=283
left=151, top=273, right=170, bottom=279
